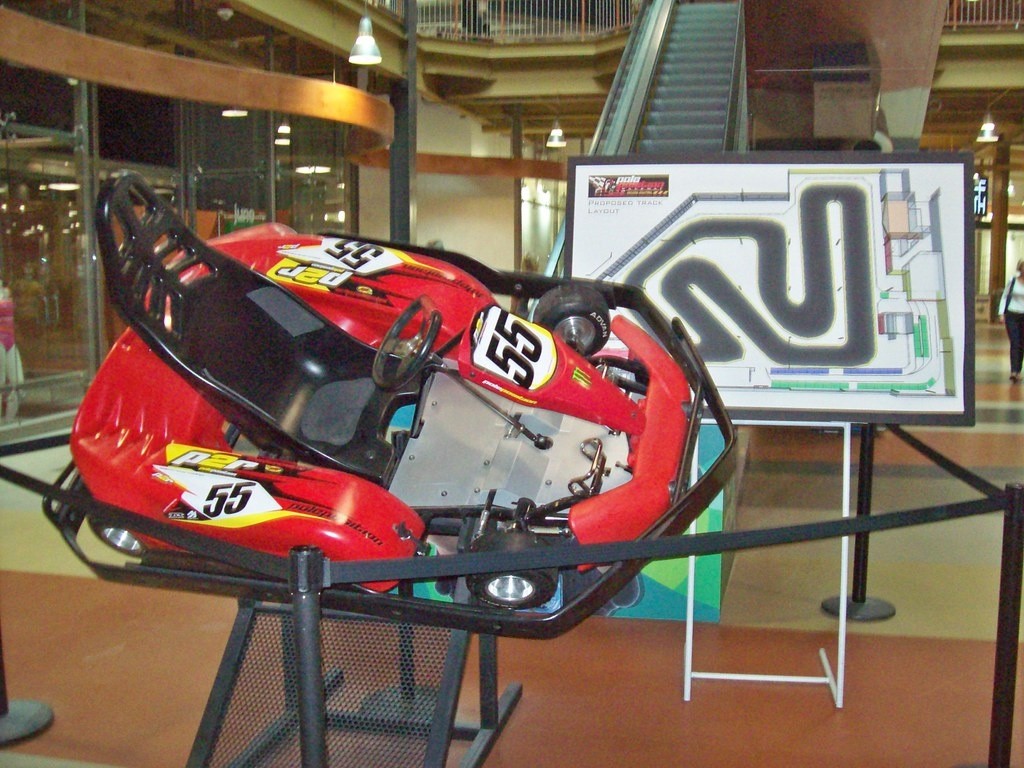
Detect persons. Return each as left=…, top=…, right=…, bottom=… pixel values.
left=15, top=261, right=45, bottom=338
left=998, top=258, right=1024, bottom=383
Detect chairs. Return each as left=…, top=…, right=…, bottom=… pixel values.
left=94, top=171, right=420, bottom=486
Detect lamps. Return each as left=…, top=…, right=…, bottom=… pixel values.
left=548, top=123, right=566, bottom=148
left=349, top=1, right=381, bottom=65
left=976, top=109, right=999, bottom=141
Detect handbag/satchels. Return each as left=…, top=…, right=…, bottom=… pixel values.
left=996, top=277, right=1015, bottom=314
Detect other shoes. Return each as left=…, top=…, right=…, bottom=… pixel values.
left=1010, top=372, right=1021, bottom=383
